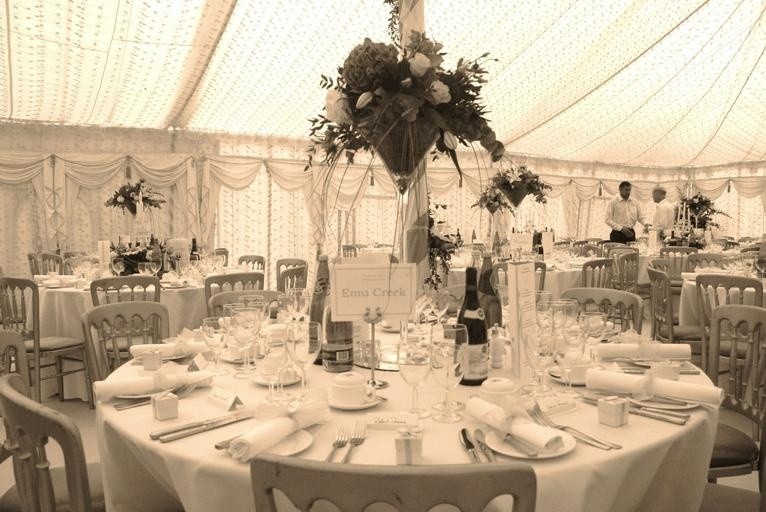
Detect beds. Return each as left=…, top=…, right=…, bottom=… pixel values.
left=245, top=449, right=540, bottom=511
left=700, top=303, right=765, bottom=482
left=697, top=414, right=765, bottom=511
left=0, top=369, right=107, bottom=510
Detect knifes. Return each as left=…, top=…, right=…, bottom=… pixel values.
left=474, top=428, right=496, bottom=463
left=584, top=395, right=691, bottom=425
left=459, top=428, right=482, bottom=465
left=150, top=410, right=244, bottom=439
left=158, top=411, right=254, bottom=443
left=621, top=370, right=701, bottom=376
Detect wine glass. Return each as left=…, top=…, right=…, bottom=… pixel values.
left=70, top=257, right=108, bottom=289
left=202, top=317, right=229, bottom=383
left=199, top=291, right=604, bottom=354
left=510, top=243, right=580, bottom=271
left=230, top=308, right=255, bottom=371
left=428, top=321, right=469, bottom=411
left=396, top=341, right=432, bottom=419
left=111, top=257, right=162, bottom=289
left=175, top=249, right=225, bottom=288
left=286, top=320, right=323, bottom=409
left=555, top=335, right=586, bottom=392
left=233, top=309, right=260, bottom=378
left=524, top=335, right=557, bottom=397
left=722, top=253, right=766, bottom=286
left=430, top=344, right=463, bottom=421
left=519, top=311, right=554, bottom=391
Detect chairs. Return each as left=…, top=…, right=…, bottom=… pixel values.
left=338, top=237, right=765, bottom=403
left=0, top=237, right=308, bottom=410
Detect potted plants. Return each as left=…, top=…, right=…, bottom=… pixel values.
left=679, top=186, right=717, bottom=216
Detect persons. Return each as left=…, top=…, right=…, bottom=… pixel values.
left=643, top=186, right=675, bottom=246
left=605, top=182, right=649, bottom=244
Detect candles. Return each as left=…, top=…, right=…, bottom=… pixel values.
left=677, top=202, right=691, bottom=223
left=135, top=191, right=150, bottom=223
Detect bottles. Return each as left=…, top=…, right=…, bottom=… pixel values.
left=456, top=229, right=461, bottom=246
left=477, top=254, right=503, bottom=328
left=670, top=231, right=677, bottom=246
left=472, top=230, right=476, bottom=243
left=492, top=232, right=500, bottom=257
left=393, top=428, right=423, bottom=465
left=190, top=239, right=200, bottom=264
left=309, top=255, right=331, bottom=367
left=456, top=267, right=488, bottom=386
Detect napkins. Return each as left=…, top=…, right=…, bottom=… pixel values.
left=466, top=396, right=567, bottom=458
left=228, top=414, right=304, bottom=463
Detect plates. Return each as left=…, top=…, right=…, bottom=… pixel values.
left=630, top=359, right=684, bottom=368
left=134, top=355, right=184, bottom=363
left=598, top=320, right=620, bottom=338
left=628, top=395, right=699, bottom=410
left=327, top=396, right=382, bottom=412
left=485, top=424, right=576, bottom=459
left=252, top=372, right=302, bottom=387
left=549, top=365, right=597, bottom=386
left=264, top=428, right=313, bottom=454
left=218, top=348, right=267, bottom=364
left=114, top=386, right=177, bottom=400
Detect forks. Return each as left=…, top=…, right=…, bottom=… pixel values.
left=525, top=402, right=623, bottom=451
left=324, top=424, right=349, bottom=463
left=340, top=414, right=368, bottom=462
left=113, top=380, right=198, bottom=410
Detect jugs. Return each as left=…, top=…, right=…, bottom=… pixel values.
left=478, top=323, right=522, bottom=393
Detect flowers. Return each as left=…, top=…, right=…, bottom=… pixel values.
left=285, top=27, right=505, bottom=193
left=490, top=164, right=551, bottom=208
left=104, top=178, right=166, bottom=218
left=471, top=180, right=515, bottom=215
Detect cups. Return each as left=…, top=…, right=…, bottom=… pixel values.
left=329, top=372, right=376, bottom=405
left=43, top=271, right=71, bottom=288
left=270, top=367, right=308, bottom=414
